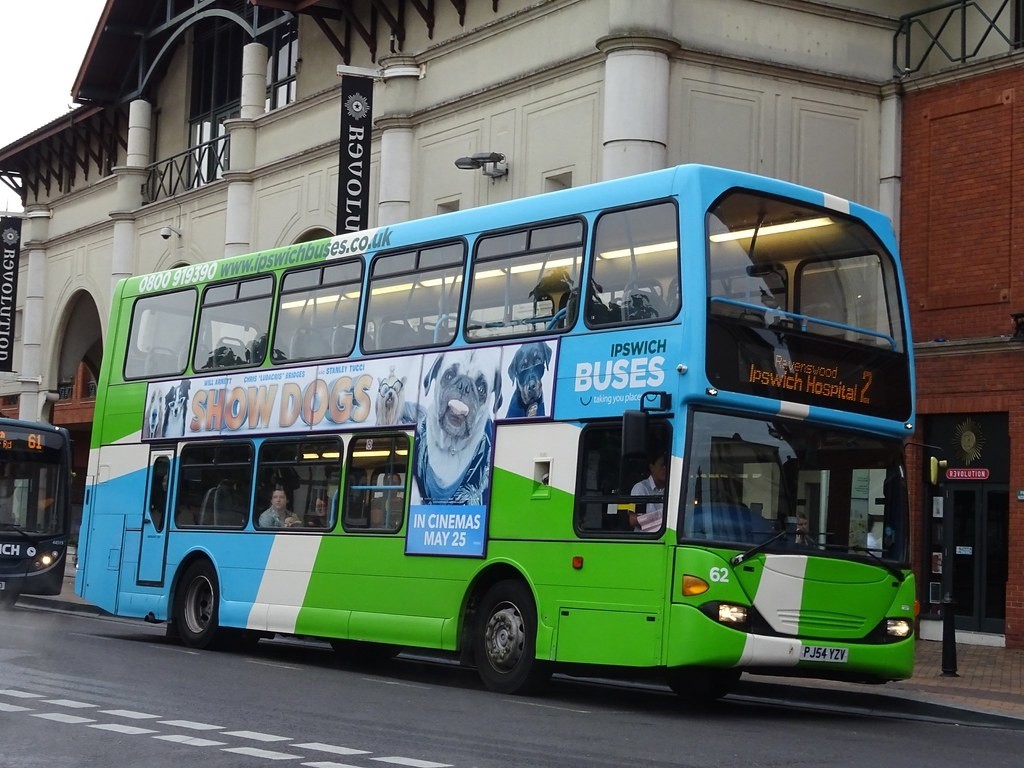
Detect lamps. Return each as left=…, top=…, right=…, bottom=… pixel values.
left=455, top=152, right=507, bottom=180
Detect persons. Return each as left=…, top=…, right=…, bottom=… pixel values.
left=373, top=473, right=403, bottom=528
left=867, top=513, right=877, bottom=548
left=259, top=489, right=299, bottom=527
left=315, top=495, right=331, bottom=515
left=796, top=511, right=814, bottom=547
left=628, top=449, right=665, bottom=532
left=284, top=516, right=302, bottom=527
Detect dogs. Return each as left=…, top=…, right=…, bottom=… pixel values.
left=415, top=350, right=501, bottom=507
left=162, top=379, right=191, bottom=438
left=375, top=376, right=424, bottom=426
left=504, top=341, right=552, bottom=418
left=143, top=389, right=165, bottom=438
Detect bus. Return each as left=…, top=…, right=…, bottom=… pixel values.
left=0, top=416, right=76, bottom=609
left=76, top=161, right=951, bottom=695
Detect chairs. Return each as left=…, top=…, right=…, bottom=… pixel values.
left=143, top=314, right=450, bottom=370
left=194, top=482, right=338, bottom=529
